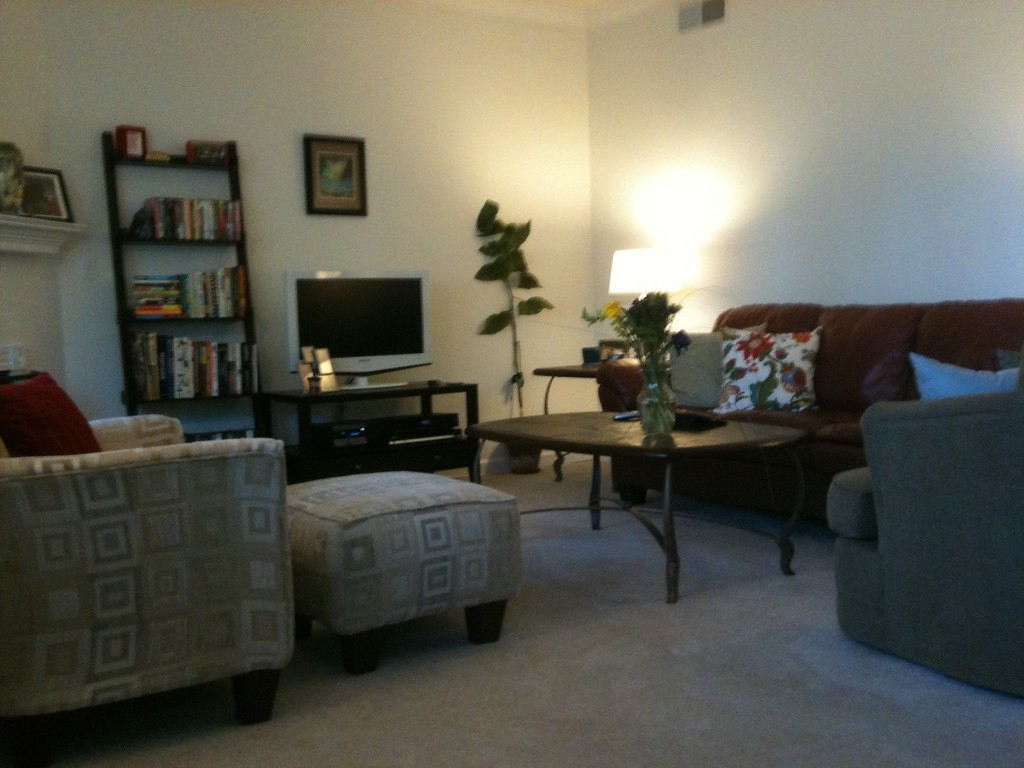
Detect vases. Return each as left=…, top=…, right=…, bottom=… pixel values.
left=636, top=354, right=679, bottom=438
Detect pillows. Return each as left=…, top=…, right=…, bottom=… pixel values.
left=0, top=372, right=103, bottom=456
left=908, top=349, right=1022, bottom=401
left=663, top=324, right=768, bottom=409
left=712, top=325, right=823, bottom=415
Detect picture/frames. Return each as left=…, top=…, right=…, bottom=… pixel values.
left=303, top=134, right=367, bottom=218
left=16, top=165, right=74, bottom=222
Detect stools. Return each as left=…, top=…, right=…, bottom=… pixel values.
left=285, top=471, right=524, bottom=674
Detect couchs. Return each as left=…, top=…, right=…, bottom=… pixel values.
left=597, top=297, right=1024, bottom=528
left=0, top=414, right=295, bottom=768
left=826, top=389, right=1024, bottom=699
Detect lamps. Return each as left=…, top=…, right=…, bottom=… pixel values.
left=607, top=247, right=683, bottom=358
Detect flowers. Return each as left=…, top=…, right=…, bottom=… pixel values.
left=579, top=291, right=692, bottom=431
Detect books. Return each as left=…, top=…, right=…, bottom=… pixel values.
left=146, top=197, right=242, bottom=243
left=124, top=332, right=262, bottom=403
left=131, top=265, right=247, bottom=320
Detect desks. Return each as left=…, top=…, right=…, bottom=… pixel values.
left=465, top=410, right=809, bottom=603
left=534, top=364, right=602, bottom=482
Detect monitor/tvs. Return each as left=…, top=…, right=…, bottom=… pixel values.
left=283, top=269, right=433, bottom=389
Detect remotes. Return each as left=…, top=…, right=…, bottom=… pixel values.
left=613, top=411, right=639, bottom=420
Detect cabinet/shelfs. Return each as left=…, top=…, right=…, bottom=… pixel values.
left=267, top=380, right=480, bottom=489
left=101, top=130, right=265, bottom=443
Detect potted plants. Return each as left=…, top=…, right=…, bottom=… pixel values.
left=478, top=201, right=558, bottom=475
